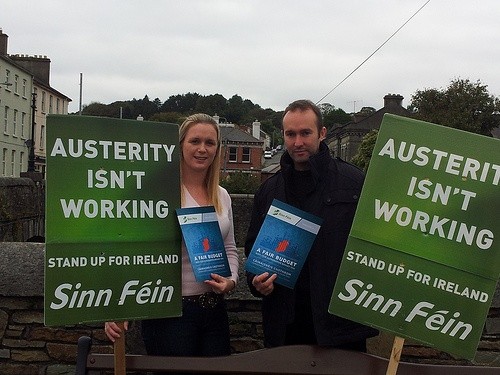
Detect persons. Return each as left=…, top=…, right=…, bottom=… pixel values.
left=243, top=99, right=381, bottom=354
left=102, top=113, right=239, bottom=358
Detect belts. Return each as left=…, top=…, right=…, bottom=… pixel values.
left=182, top=292, right=225, bottom=312
